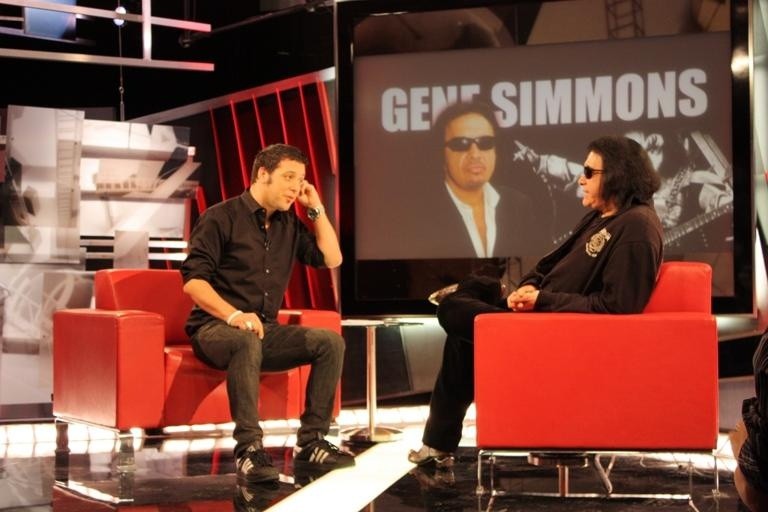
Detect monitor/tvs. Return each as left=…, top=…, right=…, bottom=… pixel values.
left=333, top=0, right=758, bottom=327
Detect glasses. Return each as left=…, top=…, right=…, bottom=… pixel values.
left=444, top=136, right=499, bottom=151
left=583, top=167, right=604, bottom=179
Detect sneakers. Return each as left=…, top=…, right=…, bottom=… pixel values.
left=292, top=439, right=353, bottom=475
left=236, top=445, right=279, bottom=484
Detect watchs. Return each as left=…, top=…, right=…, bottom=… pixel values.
left=307, top=205, right=326, bottom=221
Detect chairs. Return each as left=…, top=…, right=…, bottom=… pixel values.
left=55, top=269, right=344, bottom=461
left=473, top=261, right=718, bottom=512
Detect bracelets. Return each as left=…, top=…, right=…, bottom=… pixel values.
left=228, top=308, right=243, bottom=325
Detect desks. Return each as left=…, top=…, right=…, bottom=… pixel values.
left=342, top=318, right=424, bottom=445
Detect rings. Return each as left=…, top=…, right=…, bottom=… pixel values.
left=246, top=320, right=254, bottom=328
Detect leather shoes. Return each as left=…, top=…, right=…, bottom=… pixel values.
left=428, top=283, right=506, bottom=305
left=409, top=445, right=451, bottom=464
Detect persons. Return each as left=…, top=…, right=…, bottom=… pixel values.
left=410, top=99, right=541, bottom=256
left=231, top=467, right=329, bottom=512
left=402, top=134, right=667, bottom=470
left=512, top=126, right=732, bottom=258
left=180, top=144, right=359, bottom=484
left=408, top=466, right=463, bottom=512
